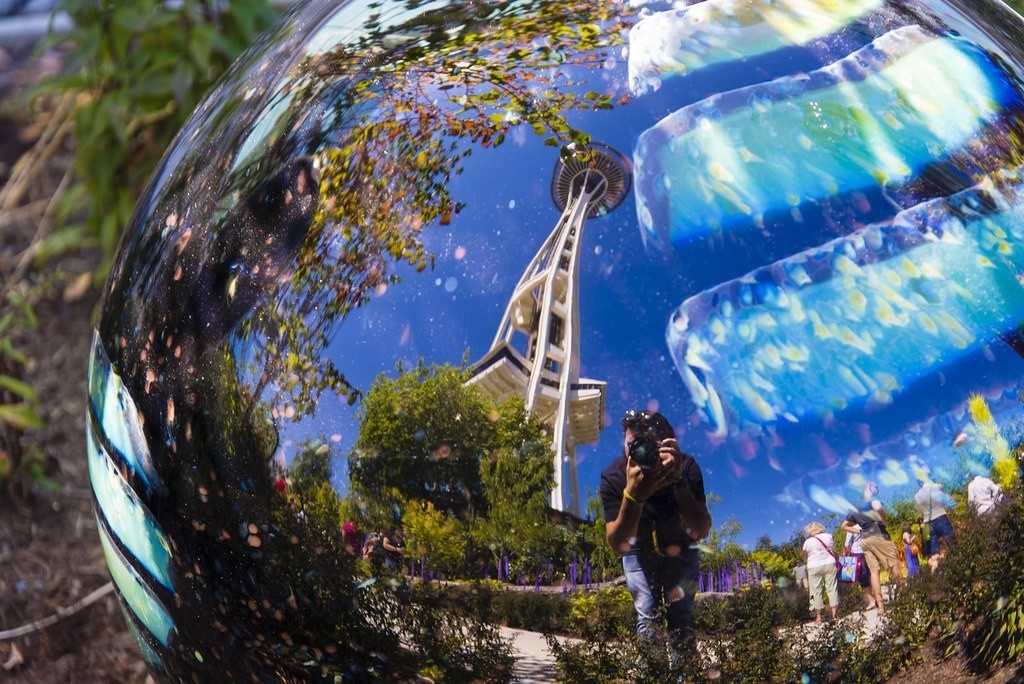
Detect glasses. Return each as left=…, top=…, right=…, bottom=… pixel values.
left=624, top=410, right=656, bottom=424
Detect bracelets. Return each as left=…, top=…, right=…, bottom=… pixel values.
left=621, top=488, right=643, bottom=505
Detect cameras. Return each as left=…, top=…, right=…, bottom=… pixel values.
left=627, top=436, right=662, bottom=469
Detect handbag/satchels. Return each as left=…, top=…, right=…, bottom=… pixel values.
left=837, top=548, right=860, bottom=584
left=834, top=557, right=843, bottom=574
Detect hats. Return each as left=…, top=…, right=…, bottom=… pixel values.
left=804, top=522, right=825, bottom=537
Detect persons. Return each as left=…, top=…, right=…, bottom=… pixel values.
left=915, top=516, right=932, bottom=557
left=342, top=517, right=359, bottom=557
left=383, top=525, right=406, bottom=576
left=800, top=521, right=838, bottom=623
left=914, top=474, right=959, bottom=571
left=846, top=511, right=876, bottom=610
left=602, top=412, right=712, bottom=666
left=967, top=472, right=999, bottom=517
left=363, top=527, right=385, bottom=569
left=901, top=525, right=919, bottom=579
left=842, top=482, right=905, bottom=616
left=995, top=483, right=1005, bottom=507
left=792, top=561, right=807, bottom=586
